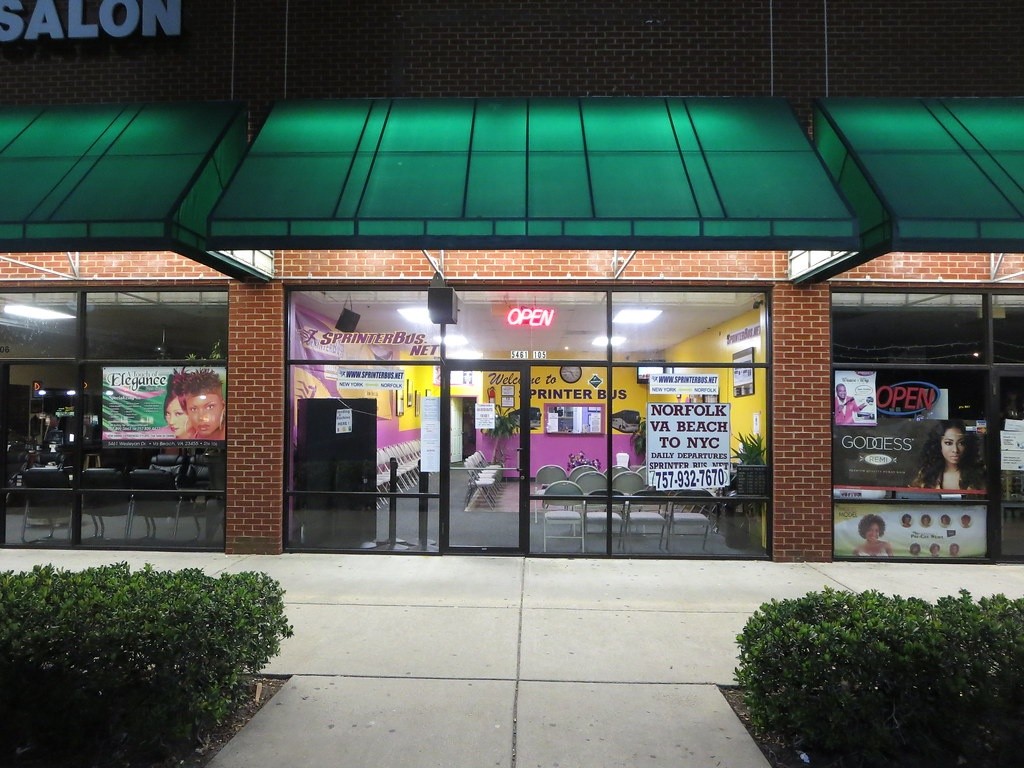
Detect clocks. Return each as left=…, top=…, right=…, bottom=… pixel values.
left=560, top=366, right=582, bottom=384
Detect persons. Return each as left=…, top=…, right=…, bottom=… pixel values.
left=929, top=543, right=940, bottom=555
left=921, top=514, right=931, bottom=528
left=853, top=514, right=894, bottom=557
left=909, top=543, right=921, bottom=555
left=940, top=514, right=951, bottom=528
left=949, top=543, right=960, bottom=555
left=960, top=515, right=971, bottom=528
left=911, top=419, right=987, bottom=491
left=901, top=513, right=912, bottom=528
left=163, top=367, right=225, bottom=440
left=835, top=383, right=874, bottom=425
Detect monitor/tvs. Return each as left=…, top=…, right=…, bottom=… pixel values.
left=636, top=359, right=665, bottom=383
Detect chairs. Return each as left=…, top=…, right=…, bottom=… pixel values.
left=533, top=464, right=716, bottom=553
left=376, top=439, right=438, bottom=510
left=463, top=450, right=505, bottom=509
left=22, top=455, right=214, bottom=542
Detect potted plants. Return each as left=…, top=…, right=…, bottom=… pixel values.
left=731, top=430, right=766, bottom=547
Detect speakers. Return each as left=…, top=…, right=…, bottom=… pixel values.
left=428, top=287, right=458, bottom=325
left=335, top=308, right=360, bottom=333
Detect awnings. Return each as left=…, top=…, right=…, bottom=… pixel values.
left=0, top=95, right=1024, bottom=286
